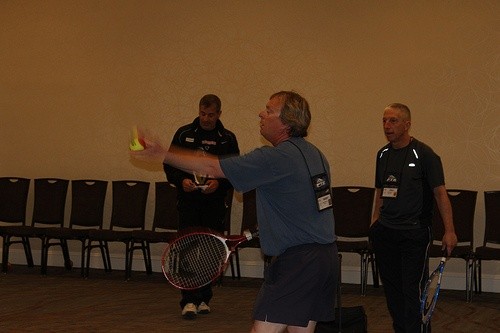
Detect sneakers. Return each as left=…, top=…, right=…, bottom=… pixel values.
left=197, top=301, right=211, bottom=315
left=182, top=302, right=198, bottom=320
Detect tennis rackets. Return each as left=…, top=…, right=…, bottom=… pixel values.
left=161, top=228, right=260, bottom=290
left=420, top=249, right=450, bottom=325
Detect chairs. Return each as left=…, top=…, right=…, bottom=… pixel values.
left=0, top=176, right=500, bottom=304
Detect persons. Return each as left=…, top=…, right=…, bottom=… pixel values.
left=162, top=93, right=240, bottom=319
left=368, top=102, right=458, bottom=333
left=131, top=90, right=336, bottom=331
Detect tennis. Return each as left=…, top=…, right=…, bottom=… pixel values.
left=129, top=135, right=146, bottom=153
